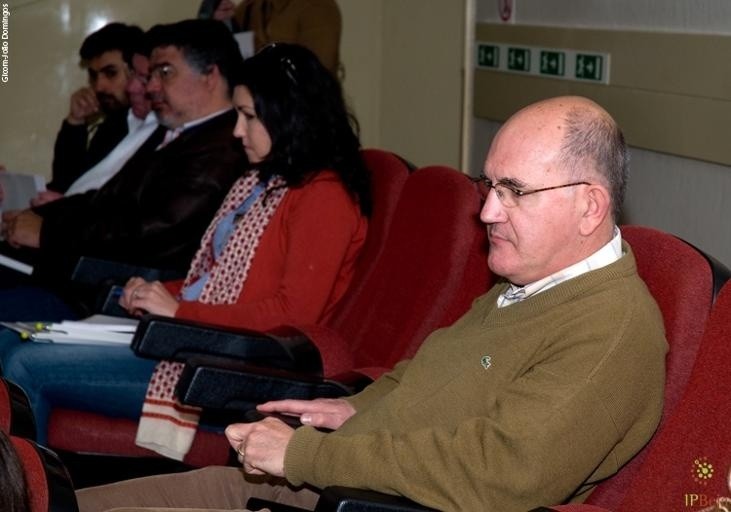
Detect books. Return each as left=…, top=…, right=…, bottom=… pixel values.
left=1, top=313, right=140, bottom=346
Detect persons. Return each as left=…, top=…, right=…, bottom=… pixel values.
left=26, top=31, right=162, bottom=208
left=29, top=21, right=146, bottom=195
left=72, top=93, right=673, bottom=512
left=0, top=41, right=375, bottom=447
left=211, top=0, right=344, bottom=79
left=0, top=17, right=250, bottom=322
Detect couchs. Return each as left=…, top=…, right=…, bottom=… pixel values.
left=3, top=146, right=729, bottom=512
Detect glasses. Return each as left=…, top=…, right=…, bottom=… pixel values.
left=475, top=178, right=591, bottom=207
left=127, top=70, right=149, bottom=90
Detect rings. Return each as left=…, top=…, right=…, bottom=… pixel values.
left=237, top=448, right=244, bottom=457
